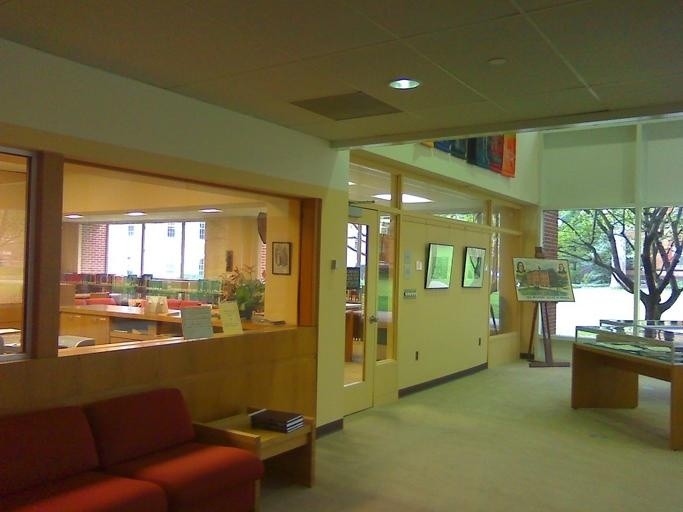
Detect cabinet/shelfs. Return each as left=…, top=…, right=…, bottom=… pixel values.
left=570, top=324, right=683, bottom=449
left=58, top=280, right=287, bottom=346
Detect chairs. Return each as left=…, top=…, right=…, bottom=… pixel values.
left=0, top=388, right=265, bottom=511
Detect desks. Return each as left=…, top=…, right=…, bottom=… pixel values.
left=192, top=402, right=317, bottom=510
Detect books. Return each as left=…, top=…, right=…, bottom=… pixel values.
left=248, top=409, right=304, bottom=433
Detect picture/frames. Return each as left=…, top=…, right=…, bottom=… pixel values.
left=424, top=242, right=487, bottom=289
left=271, top=241, right=293, bottom=276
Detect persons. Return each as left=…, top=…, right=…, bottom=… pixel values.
left=516, top=262, right=526, bottom=273
left=558, top=264, right=567, bottom=274
